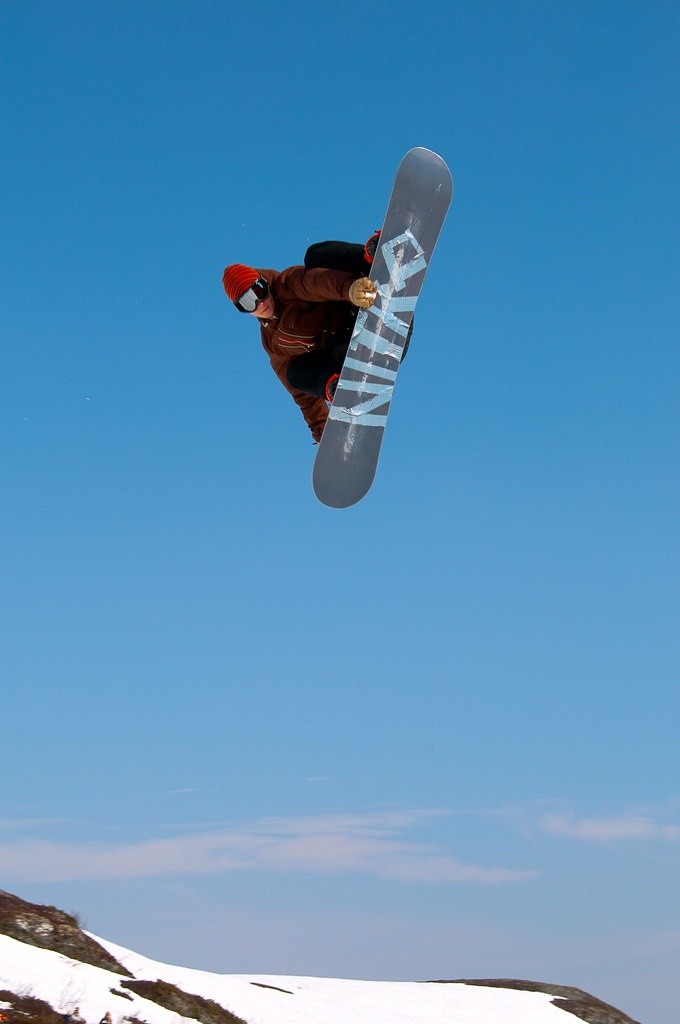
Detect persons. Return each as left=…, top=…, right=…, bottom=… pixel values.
left=223, top=232, right=414, bottom=444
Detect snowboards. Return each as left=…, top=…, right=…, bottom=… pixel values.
left=310, top=146, right=455, bottom=511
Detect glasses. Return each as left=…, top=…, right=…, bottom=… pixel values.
left=234, top=275, right=269, bottom=314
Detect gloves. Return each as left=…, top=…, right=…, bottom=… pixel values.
left=349, top=278, right=378, bottom=309
left=312, top=424, right=325, bottom=444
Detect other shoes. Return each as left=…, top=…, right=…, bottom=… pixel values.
left=325, top=373, right=339, bottom=402
left=364, top=229, right=382, bottom=263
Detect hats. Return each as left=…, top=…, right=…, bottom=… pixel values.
left=222, top=263, right=259, bottom=305
